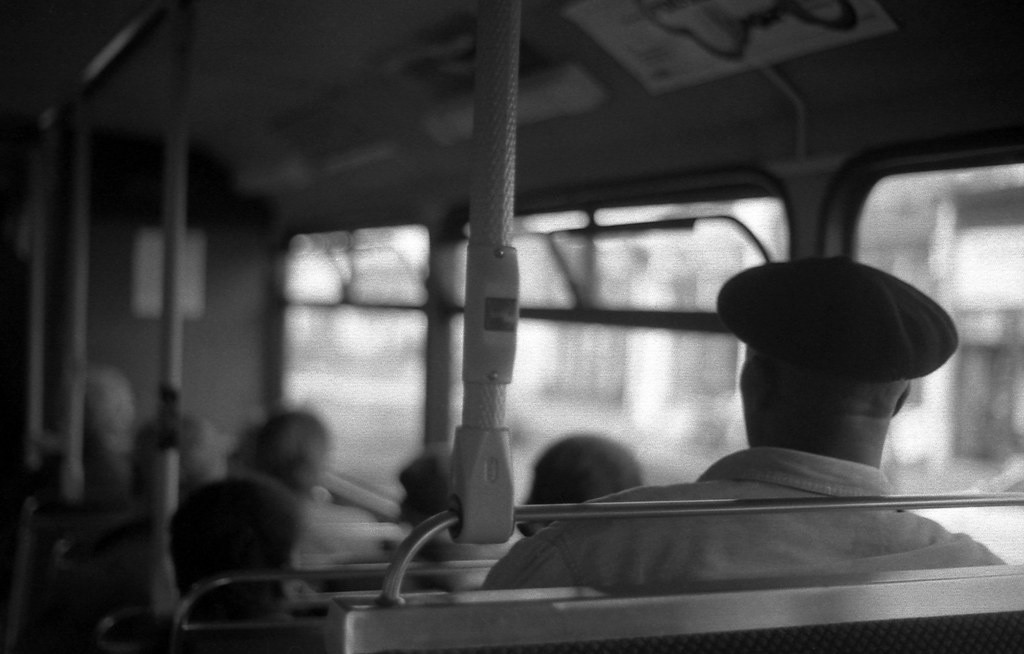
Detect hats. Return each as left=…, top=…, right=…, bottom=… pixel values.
left=169, top=475, right=305, bottom=579
left=516, top=432, right=649, bottom=538
left=717, top=256, right=959, bottom=386
left=234, top=411, right=332, bottom=482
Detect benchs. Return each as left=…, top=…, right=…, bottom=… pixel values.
left=170, top=560, right=501, bottom=654
left=327, top=490, right=1024, bottom=654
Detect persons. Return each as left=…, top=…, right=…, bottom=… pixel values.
left=517, top=435, right=644, bottom=537
left=483, top=256, right=1007, bottom=592
left=1, top=363, right=464, bottom=654
